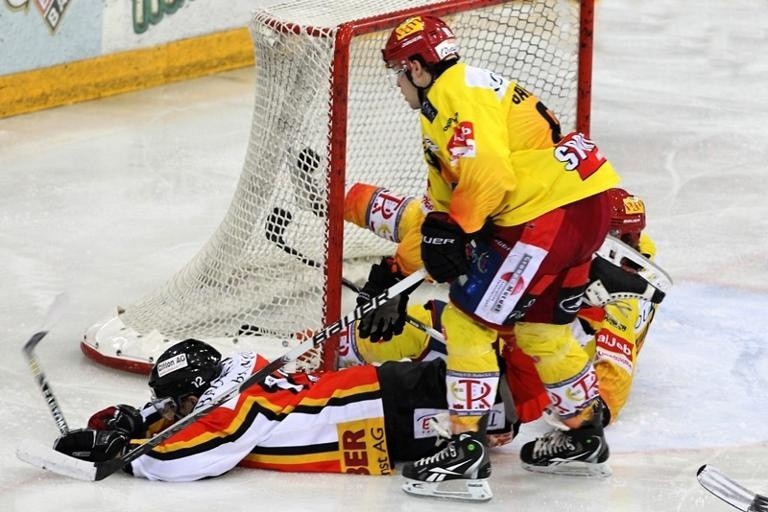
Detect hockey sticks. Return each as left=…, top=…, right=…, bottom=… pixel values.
left=265, top=208, right=446, bottom=346
left=17, top=266, right=428, bottom=481
left=23, top=330, right=69, bottom=436
left=697, top=464, right=768, bottom=512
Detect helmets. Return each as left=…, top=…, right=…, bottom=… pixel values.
left=148, top=338, right=221, bottom=398
left=605, top=188, right=646, bottom=239
left=381, top=14, right=461, bottom=63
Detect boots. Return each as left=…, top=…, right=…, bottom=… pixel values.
left=558, top=252, right=666, bottom=315
left=401, top=432, right=494, bottom=482
left=298, top=148, right=326, bottom=217
left=520, top=430, right=610, bottom=467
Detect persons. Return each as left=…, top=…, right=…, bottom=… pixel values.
left=235, top=145, right=658, bottom=436
left=354, top=11, right=623, bottom=482
left=52, top=253, right=667, bottom=484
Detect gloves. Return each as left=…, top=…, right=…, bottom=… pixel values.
left=420, top=213, right=476, bottom=284
left=356, top=263, right=409, bottom=343
left=52, top=403, right=147, bottom=462
